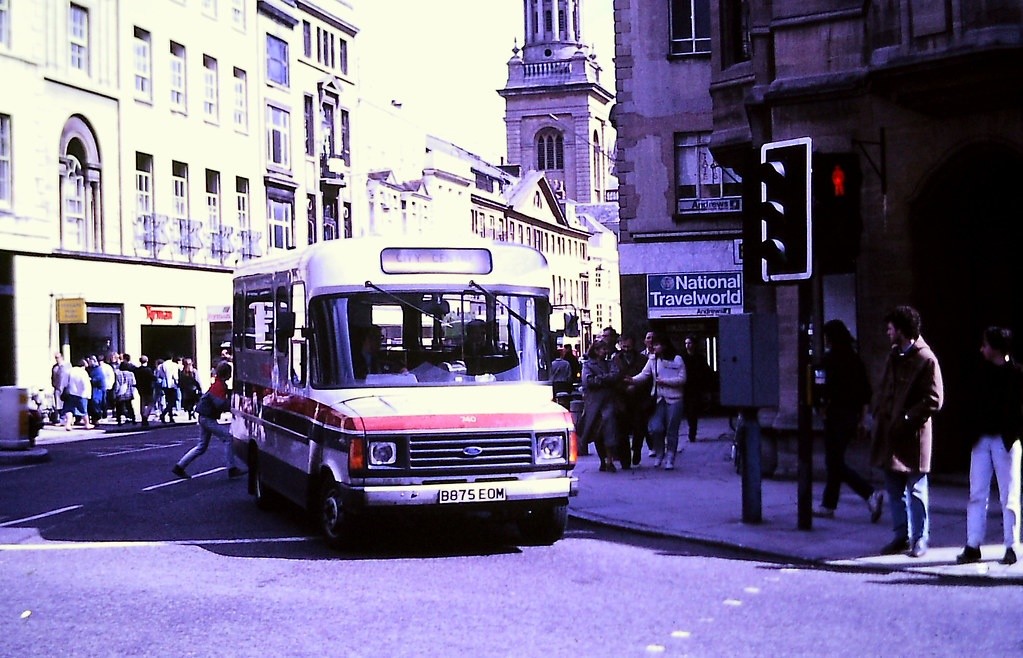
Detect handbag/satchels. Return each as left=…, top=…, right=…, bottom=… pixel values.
left=637, top=394, right=657, bottom=417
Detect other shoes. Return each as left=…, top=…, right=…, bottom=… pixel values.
left=661, top=451, right=676, bottom=469
left=171, top=464, right=192, bottom=479
left=997, top=548, right=1017, bottom=567
left=606, top=462, right=616, bottom=472
left=653, top=458, right=662, bottom=466
left=631, top=449, right=642, bottom=465
left=956, top=544, right=981, bottom=563
left=865, top=491, right=884, bottom=524
left=44, top=410, right=196, bottom=430
left=688, top=434, right=696, bottom=442
left=812, top=505, right=834, bottom=518
left=880, top=538, right=912, bottom=555
left=621, top=462, right=631, bottom=469
left=598, top=463, right=607, bottom=471
left=648, top=449, right=656, bottom=457
left=911, top=538, right=928, bottom=557
left=227, top=468, right=250, bottom=480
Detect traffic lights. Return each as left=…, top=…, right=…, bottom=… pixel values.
left=755, top=137, right=812, bottom=283
left=812, top=151, right=863, bottom=249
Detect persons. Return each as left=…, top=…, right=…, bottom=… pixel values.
left=452, top=319, right=505, bottom=359
left=350, top=323, right=407, bottom=379
left=867, top=305, right=943, bottom=557
left=576, top=326, right=702, bottom=470
left=550, top=343, right=582, bottom=399
left=953, top=325, right=1023, bottom=564
left=48, top=350, right=201, bottom=430
left=172, top=361, right=250, bottom=480
left=812, top=320, right=884, bottom=524
left=210, top=351, right=235, bottom=398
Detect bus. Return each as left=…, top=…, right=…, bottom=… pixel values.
left=229, top=229, right=581, bottom=551
